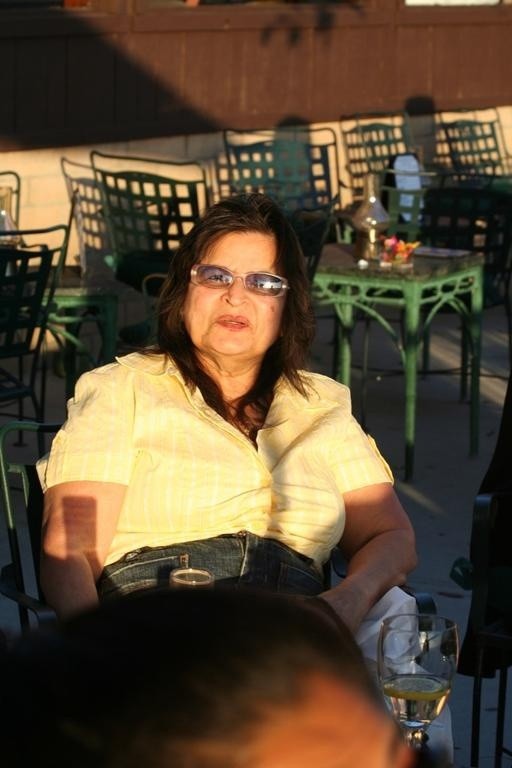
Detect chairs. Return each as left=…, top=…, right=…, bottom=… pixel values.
left=448, top=490, right=511, bottom=768
left=1, top=418, right=353, bottom=639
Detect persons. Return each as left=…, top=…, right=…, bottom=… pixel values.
left=40, top=197, right=417, bottom=642
left=0, top=577, right=411, bottom=768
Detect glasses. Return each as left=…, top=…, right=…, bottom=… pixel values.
left=191, top=264, right=291, bottom=299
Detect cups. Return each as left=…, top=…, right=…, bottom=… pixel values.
left=170, top=568, right=214, bottom=592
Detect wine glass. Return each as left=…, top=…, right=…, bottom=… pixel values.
left=375, top=614, right=459, bottom=747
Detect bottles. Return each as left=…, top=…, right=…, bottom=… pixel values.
left=0, top=186, right=22, bottom=277
left=353, top=172, right=391, bottom=260
